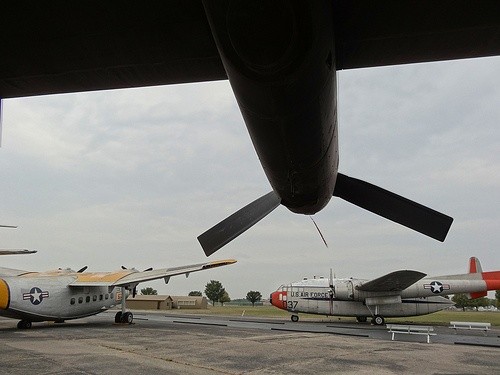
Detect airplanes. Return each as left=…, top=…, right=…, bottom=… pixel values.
left=270, top=257, right=500, bottom=326
left=0, top=0, right=499, bottom=258
left=0, top=259, right=238, bottom=329
